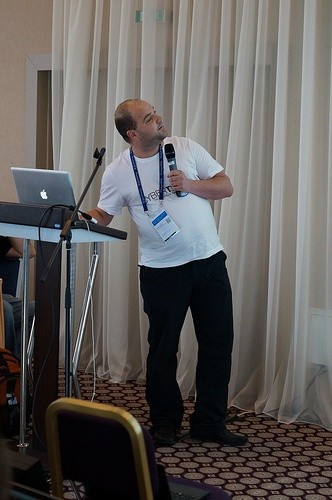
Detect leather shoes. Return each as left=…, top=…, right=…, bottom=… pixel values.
left=149, top=425, right=183, bottom=446
left=190, top=427, right=247, bottom=446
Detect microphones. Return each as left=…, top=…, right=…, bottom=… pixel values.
left=165, top=143, right=181, bottom=197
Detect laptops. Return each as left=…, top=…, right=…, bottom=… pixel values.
left=11, top=167, right=76, bottom=208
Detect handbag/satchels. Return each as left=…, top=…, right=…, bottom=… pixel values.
left=0, top=348, right=30, bottom=406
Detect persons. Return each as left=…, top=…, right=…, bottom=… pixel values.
left=0, top=235, right=36, bottom=359
left=78, top=99, right=248, bottom=449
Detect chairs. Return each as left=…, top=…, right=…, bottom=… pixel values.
left=44, top=397, right=230, bottom=500
